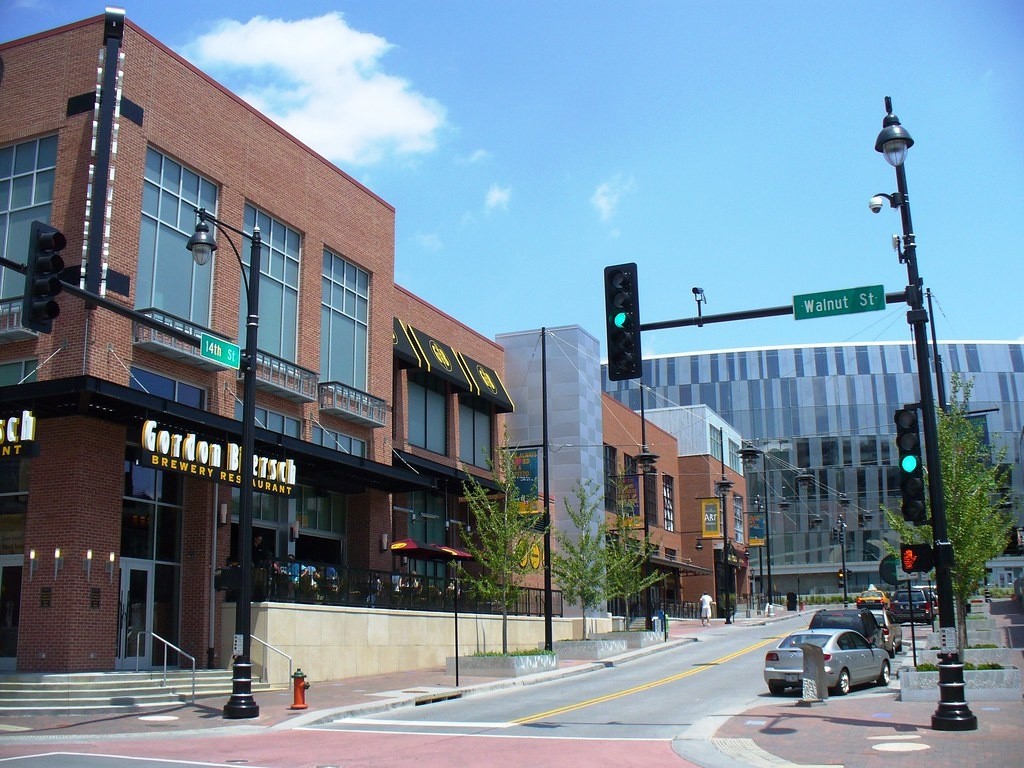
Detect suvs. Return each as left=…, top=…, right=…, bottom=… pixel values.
left=888, top=585, right=939, bottom=625
left=808, top=608, right=889, bottom=650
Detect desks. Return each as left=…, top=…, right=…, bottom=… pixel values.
left=272, top=574, right=298, bottom=602
left=402, top=586, right=420, bottom=608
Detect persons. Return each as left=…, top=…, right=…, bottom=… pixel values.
left=699, top=592, right=712, bottom=627
left=366, top=566, right=461, bottom=609
left=225, top=555, right=348, bottom=601
left=252, top=534, right=269, bottom=600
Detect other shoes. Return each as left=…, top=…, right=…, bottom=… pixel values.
left=702, top=624, right=705, bottom=626
left=707, top=623, right=711, bottom=626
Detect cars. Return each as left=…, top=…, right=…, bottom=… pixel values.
left=765, top=628, right=891, bottom=693
left=856, top=584, right=890, bottom=610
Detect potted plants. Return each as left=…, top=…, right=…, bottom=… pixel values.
left=953, top=596, right=990, bottom=613
left=934, top=614, right=996, bottom=631
left=917, top=644, right=1022, bottom=665
left=926, top=627, right=1002, bottom=648
left=897, top=661, right=1022, bottom=702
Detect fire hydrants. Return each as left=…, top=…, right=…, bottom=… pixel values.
left=291, top=668, right=311, bottom=711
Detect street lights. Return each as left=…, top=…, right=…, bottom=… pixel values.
left=186, top=206, right=262, bottom=718
left=743, top=453, right=775, bottom=618
left=695, top=426, right=733, bottom=625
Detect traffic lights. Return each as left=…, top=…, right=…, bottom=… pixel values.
left=602, top=261, right=643, bottom=383
left=847, top=568, right=852, bottom=580
left=894, top=408, right=928, bottom=522
left=900, top=543, right=933, bottom=573
left=839, top=570, right=844, bottom=581
left=22, top=220, right=67, bottom=335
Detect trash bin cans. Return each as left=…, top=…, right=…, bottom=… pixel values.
left=786, top=592, right=796, bottom=611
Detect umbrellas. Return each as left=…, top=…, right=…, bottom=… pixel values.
left=387, top=536, right=473, bottom=563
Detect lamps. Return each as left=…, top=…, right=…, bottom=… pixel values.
left=289, top=520, right=300, bottom=542
left=379, top=533, right=388, bottom=554
left=105, top=551, right=116, bottom=583
left=54, top=548, right=65, bottom=582
left=82, top=549, right=93, bottom=583
left=29, top=549, right=39, bottom=583
left=217, top=503, right=228, bottom=528
left=400, top=556, right=408, bottom=567
